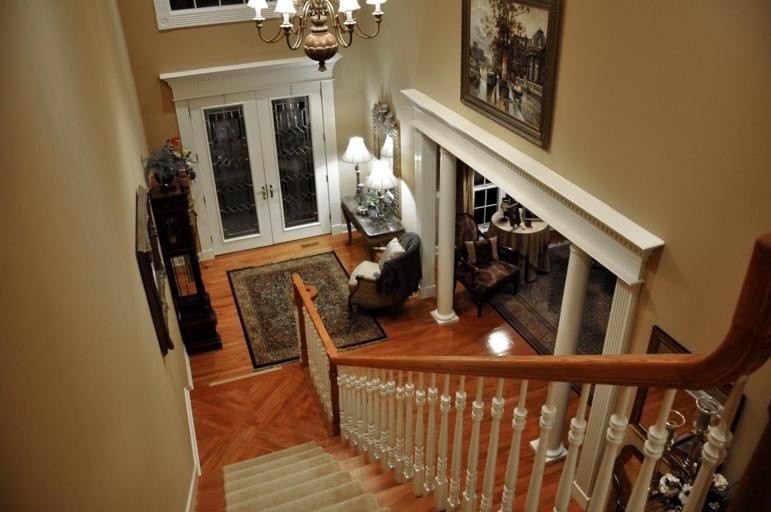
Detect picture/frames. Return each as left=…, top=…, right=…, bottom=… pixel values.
left=460, top=0, right=562, bottom=150
left=134, top=185, right=175, bottom=355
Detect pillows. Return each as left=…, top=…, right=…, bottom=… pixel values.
left=464, top=236, right=499, bottom=264
left=378, top=237, right=406, bottom=270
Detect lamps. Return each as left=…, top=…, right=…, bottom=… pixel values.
left=246, top=0, right=388, bottom=72
left=366, top=160, right=394, bottom=228
left=342, top=136, right=372, bottom=202
left=379, top=134, right=393, bottom=158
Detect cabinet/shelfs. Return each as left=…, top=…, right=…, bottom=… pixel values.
left=149, top=179, right=222, bottom=356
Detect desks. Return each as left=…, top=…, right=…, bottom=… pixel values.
left=488, top=210, right=552, bottom=285
left=341, top=198, right=406, bottom=247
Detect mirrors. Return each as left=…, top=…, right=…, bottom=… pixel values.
left=371, top=102, right=401, bottom=218
left=628, top=324, right=746, bottom=486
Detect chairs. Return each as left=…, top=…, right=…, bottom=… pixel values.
left=454, top=212, right=520, bottom=317
left=347, top=231, right=420, bottom=312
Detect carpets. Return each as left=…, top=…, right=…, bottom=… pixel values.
left=225, top=250, right=392, bottom=370
left=490, top=255, right=613, bottom=408
